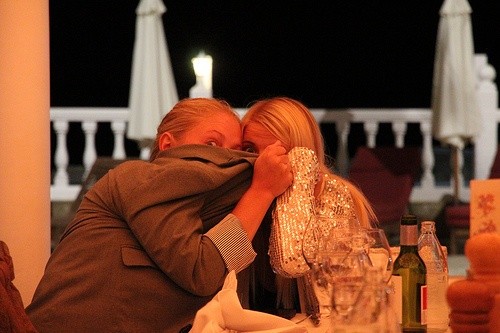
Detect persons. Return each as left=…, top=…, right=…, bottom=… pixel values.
left=237, top=96, right=386, bottom=325
left=21, top=95, right=294, bottom=333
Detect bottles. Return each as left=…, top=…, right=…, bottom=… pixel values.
left=417, top=220, right=450, bottom=332
left=347, top=264, right=401, bottom=332
left=391, top=215, right=427, bottom=333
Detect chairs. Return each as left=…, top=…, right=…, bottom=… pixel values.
left=344, top=146, right=421, bottom=244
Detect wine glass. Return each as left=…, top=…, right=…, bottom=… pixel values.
left=302, top=214, right=392, bottom=315
